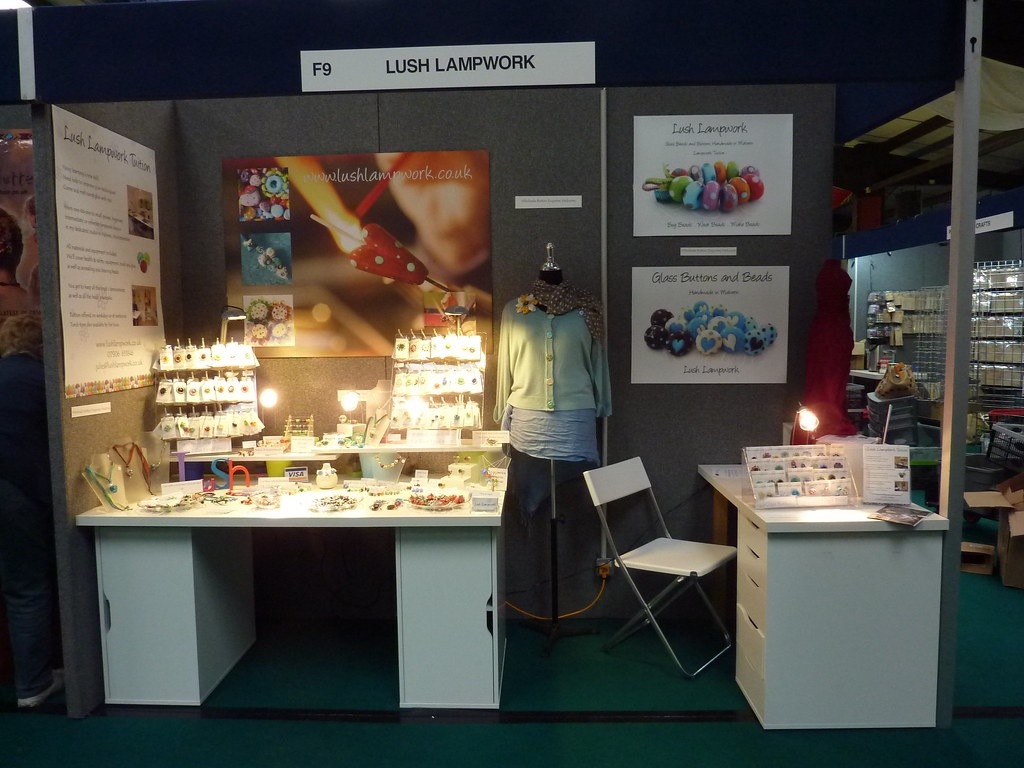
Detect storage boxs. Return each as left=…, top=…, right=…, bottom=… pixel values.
left=849, top=338, right=867, bottom=371
left=846, top=379, right=1024, bottom=586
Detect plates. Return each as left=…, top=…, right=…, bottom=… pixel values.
left=311, top=495, right=366, bottom=510
left=137, top=502, right=190, bottom=512
left=413, top=504, right=455, bottom=510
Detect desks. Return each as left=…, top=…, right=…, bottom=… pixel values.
left=850, top=370, right=928, bottom=383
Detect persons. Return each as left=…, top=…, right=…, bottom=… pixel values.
left=493, top=261, right=612, bottom=513
left=0, top=314, right=68, bottom=708
left=0, top=205, right=42, bottom=314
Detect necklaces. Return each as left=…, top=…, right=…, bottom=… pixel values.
left=85, top=461, right=120, bottom=492
left=112, top=442, right=135, bottom=478
left=372, top=390, right=392, bottom=424
left=1, top=280, right=22, bottom=290
left=150, top=441, right=167, bottom=470
left=375, top=455, right=401, bottom=469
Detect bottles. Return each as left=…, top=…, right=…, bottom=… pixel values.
left=316, top=463, right=338, bottom=489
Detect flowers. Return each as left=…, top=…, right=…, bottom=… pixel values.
left=515, top=292, right=538, bottom=314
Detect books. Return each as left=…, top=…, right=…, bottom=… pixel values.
left=863, top=446, right=911, bottom=504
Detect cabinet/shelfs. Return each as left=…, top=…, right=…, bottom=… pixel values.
left=76, top=439, right=511, bottom=709
left=698, top=464, right=950, bottom=730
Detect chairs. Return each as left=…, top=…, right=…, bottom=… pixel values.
left=583, top=456, right=738, bottom=680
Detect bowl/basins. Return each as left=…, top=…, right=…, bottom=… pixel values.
left=250, top=489, right=297, bottom=510
